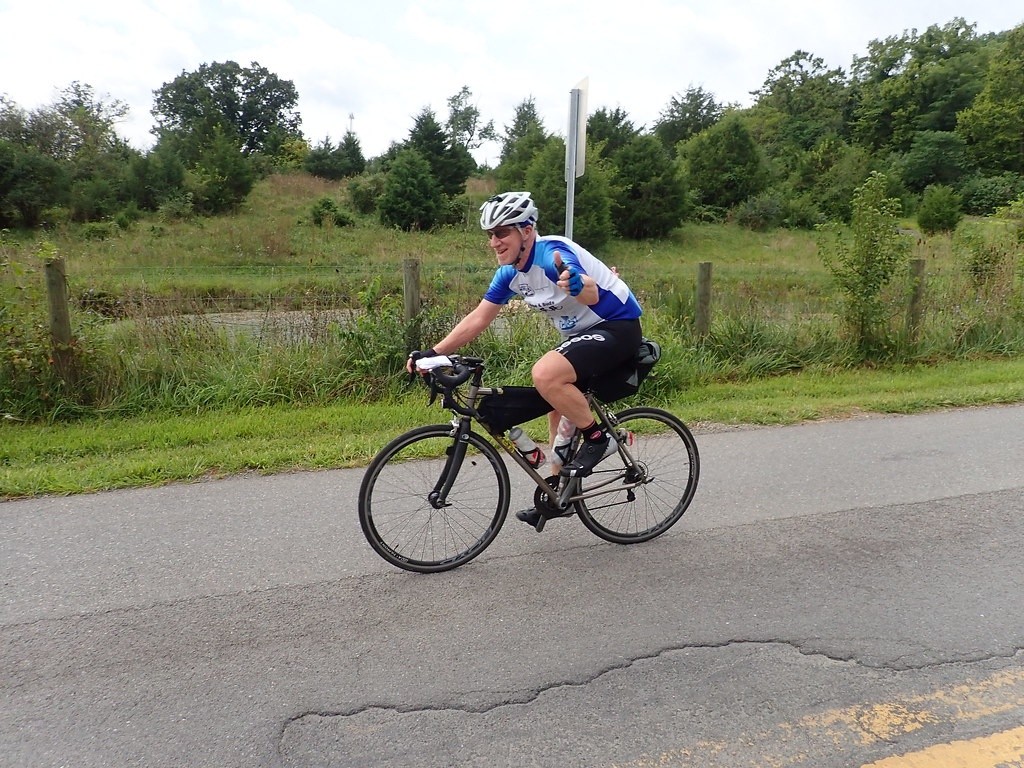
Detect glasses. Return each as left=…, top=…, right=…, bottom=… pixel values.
left=487, top=227, right=516, bottom=239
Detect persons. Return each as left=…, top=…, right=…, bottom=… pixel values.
left=406, top=192, right=642, bottom=524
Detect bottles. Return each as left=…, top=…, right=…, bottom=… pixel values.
left=508, top=426, right=547, bottom=469
left=552, top=414, right=576, bottom=464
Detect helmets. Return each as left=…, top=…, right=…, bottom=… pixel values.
left=479, top=191, right=539, bottom=231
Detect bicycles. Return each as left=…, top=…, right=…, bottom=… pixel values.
left=357, top=352, right=702, bottom=574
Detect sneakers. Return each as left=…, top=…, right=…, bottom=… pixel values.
left=560, top=432, right=618, bottom=477
left=516, top=502, right=573, bottom=524
